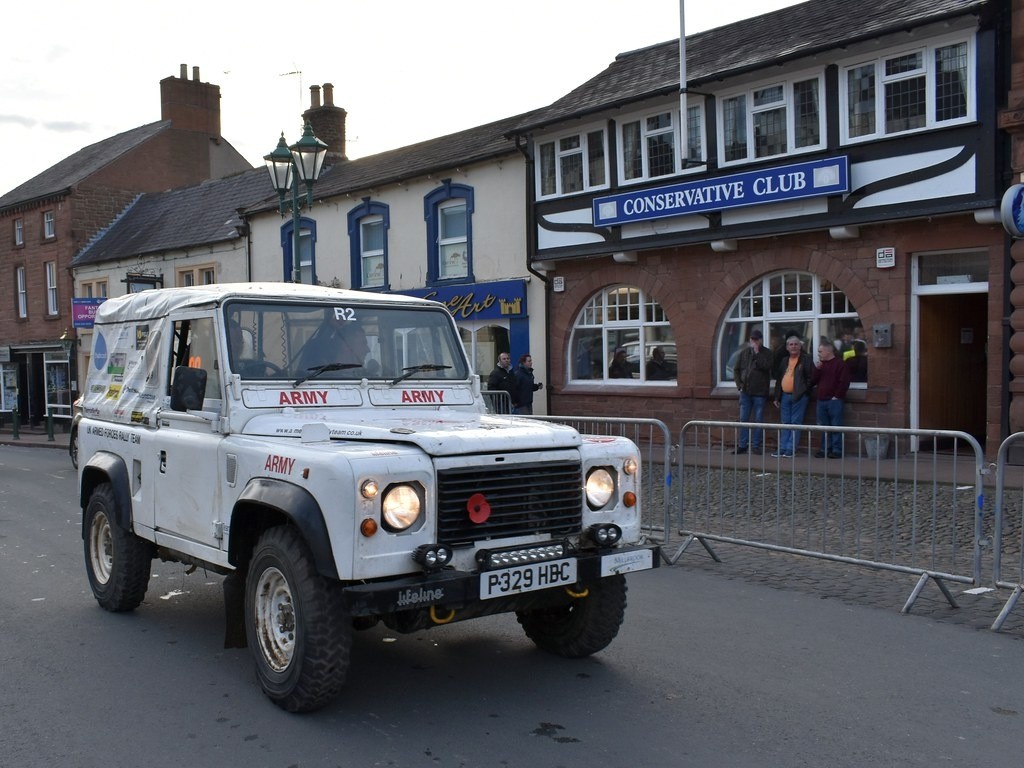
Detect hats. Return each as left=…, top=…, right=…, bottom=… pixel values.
left=750, top=330, right=762, bottom=339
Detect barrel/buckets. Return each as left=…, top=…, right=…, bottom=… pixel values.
left=865, top=438, right=889, bottom=460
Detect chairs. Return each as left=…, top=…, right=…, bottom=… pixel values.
left=295, top=338, right=337, bottom=379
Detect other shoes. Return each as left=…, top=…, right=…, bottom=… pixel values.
left=732, top=447, right=747, bottom=454
left=815, top=452, right=824, bottom=457
left=783, top=450, right=798, bottom=457
left=753, top=448, right=762, bottom=454
left=828, top=452, right=842, bottom=458
left=771, top=449, right=785, bottom=457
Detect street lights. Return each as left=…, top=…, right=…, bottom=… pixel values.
left=263, top=119, right=328, bottom=282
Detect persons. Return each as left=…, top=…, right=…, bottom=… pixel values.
left=510, top=355, right=543, bottom=415
left=487, top=352, right=523, bottom=414
left=609, top=350, right=636, bottom=378
left=195, top=316, right=267, bottom=380
left=813, top=342, right=851, bottom=458
left=644, top=347, right=674, bottom=380
left=771, top=336, right=819, bottom=458
left=730, top=330, right=778, bottom=455
left=311, top=320, right=380, bottom=379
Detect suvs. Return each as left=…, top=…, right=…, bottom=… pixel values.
left=79, top=281, right=661, bottom=715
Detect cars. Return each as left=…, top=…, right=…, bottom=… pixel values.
left=608, top=341, right=736, bottom=381
